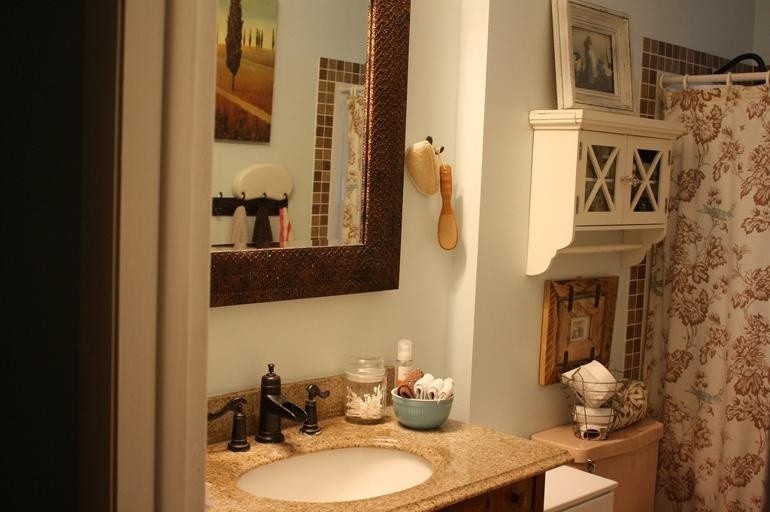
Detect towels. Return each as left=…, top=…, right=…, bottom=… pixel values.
left=252, top=207, right=273, bottom=249
left=397, top=368, right=454, bottom=404
left=230, top=205, right=250, bottom=250
left=278, top=207, right=292, bottom=249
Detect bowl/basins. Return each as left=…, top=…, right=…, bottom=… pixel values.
left=391, top=386, right=454, bottom=431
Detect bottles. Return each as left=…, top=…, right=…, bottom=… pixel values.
left=343, top=355, right=387, bottom=425
left=393, top=339, right=414, bottom=390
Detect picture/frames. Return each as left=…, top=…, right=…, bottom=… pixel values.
left=551, top=0, right=634, bottom=116
left=540, top=276, right=620, bottom=387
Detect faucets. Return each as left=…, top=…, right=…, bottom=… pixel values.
left=255, top=362, right=310, bottom=444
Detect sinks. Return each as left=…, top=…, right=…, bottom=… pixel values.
left=227, top=439, right=436, bottom=506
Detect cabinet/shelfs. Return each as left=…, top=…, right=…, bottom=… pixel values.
left=525, top=109, right=685, bottom=279
left=436, top=472, right=544, bottom=512
left=544, top=465, right=619, bottom=512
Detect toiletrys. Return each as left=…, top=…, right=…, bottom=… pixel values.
left=394, top=339, right=413, bottom=387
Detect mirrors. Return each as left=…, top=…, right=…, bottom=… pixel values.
left=210, top=0, right=412, bottom=309
